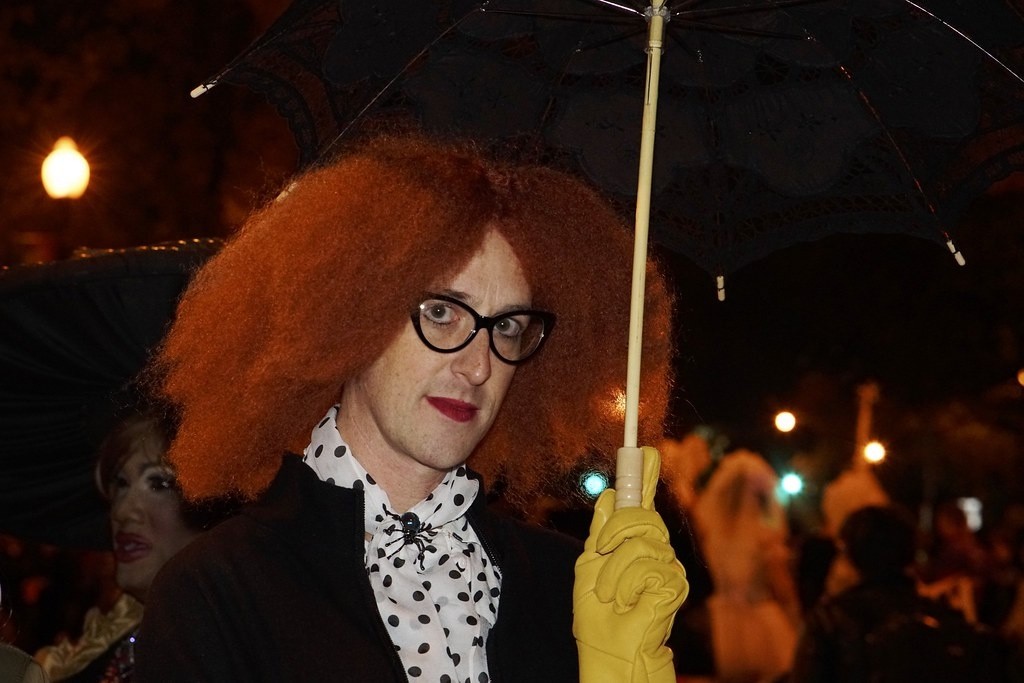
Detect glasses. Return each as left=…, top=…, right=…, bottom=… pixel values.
left=409, top=291, right=556, bottom=365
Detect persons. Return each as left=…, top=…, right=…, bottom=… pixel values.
left=136, top=138, right=691, bottom=683
left=660, top=416, right=1024, bottom=683
left=0, top=377, right=265, bottom=683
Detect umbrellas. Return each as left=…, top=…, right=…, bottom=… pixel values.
left=191, top=0, right=1024, bottom=513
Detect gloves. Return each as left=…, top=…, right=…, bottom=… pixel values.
left=572, top=445, right=689, bottom=683
